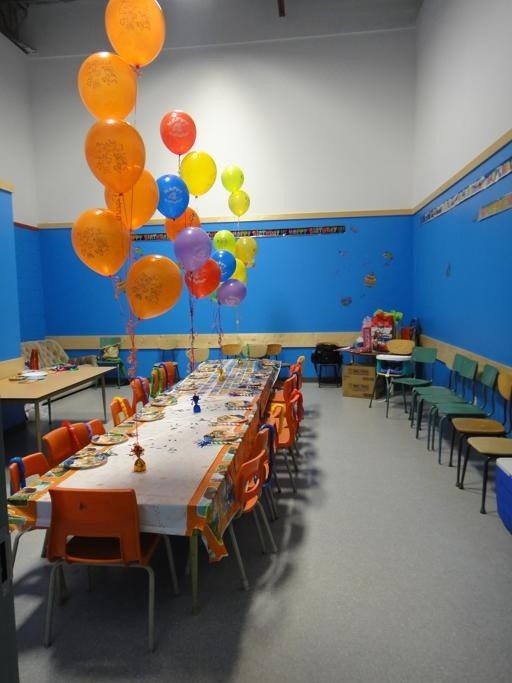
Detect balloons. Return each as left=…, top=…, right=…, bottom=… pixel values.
left=178, top=151, right=217, bottom=198
left=154, top=174, right=190, bottom=223
left=105, top=170, right=160, bottom=235
left=221, top=165, right=244, bottom=194
left=104, top=0, right=165, bottom=72
left=77, top=52, right=137, bottom=120
left=215, top=229, right=234, bottom=253
left=232, top=235, right=258, bottom=263
left=227, top=258, right=246, bottom=282
left=174, top=226, right=212, bottom=276
left=125, top=255, right=183, bottom=324
left=226, top=189, right=249, bottom=216
left=84, top=118, right=146, bottom=198
left=165, top=208, right=200, bottom=245
left=161, top=110, right=196, bottom=156
left=207, top=250, right=237, bottom=285
left=207, top=284, right=223, bottom=301
left=183, top=258, right=222, bottom=300
left=72, top=208, right=129, bottom=280
left=217, top=279, right=246, bottom=306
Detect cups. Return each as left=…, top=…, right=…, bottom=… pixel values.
left=247, top=359, right=256, bottom=372
left=58, top=365, right=64, bottom=374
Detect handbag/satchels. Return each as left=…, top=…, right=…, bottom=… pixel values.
left=311, top=343, right=343, bottom=382
left=100, top=343, right=120, bottom=361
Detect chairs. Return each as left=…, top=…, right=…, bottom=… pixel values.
left=13, top=452, right=50, bottom=566
left=69, top=423, right=93, bottom=453
left=258, top=423, right=279, bottom=522
left=164, top=361, right=176, bottom=389
left=267, top=344, right=282, bottom=360
left=94, top=337, right=122, bottom=390
left=272, top=365, right=300, bottom=441
left=369, top=339, right=414, bottom=418
left=460, top=437, right=512, bottom=515
left=44, top=489, right=181, bottom=651
left=243, top=344, right=267, bottom=358
left=384, top=344, right=438, bottom=419
left=316, top=342, right=340, bottom=388
left=42, top=426, right=76, bottom=469
left=110, top=399, right=133, bottom=426
left=277, top=355, right=305, bottom=423
left=451, top=370, right=512, bottom=485
left=243, top=428, right=276, bottom=552
left=275, top=395, right=300, bottom=491
left=130, top=378, right=151, bottom=412
left=266, top=375, right=300, bottom=472
left=196, top=452, right=277, bottom=591
left=185, top=347, right=209, bottom=363
left=416, top=358, right=479, bottom=450
left=151, top=365, right=168, bottom=400
left=87, top=418, right=106, bottom=437
left=411, top=351, right=464, bottom=431
left=221, top=344, right=242, bottom=359
left=432, top=365, right=500, bottom=468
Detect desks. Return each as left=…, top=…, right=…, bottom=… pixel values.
left=335, top=343, right=387, bottom=366
left=0, top=356, right=118, bottom=452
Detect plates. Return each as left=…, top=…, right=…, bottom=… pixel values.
left=150, top=399, right=179, bottom=407
left=226, top=400, right=252, bottom=409
left=20, top=371, right=49, bottom=379
left=132, top=412, right=167, bottom=422
left=66, top=451, right=109, bottom=468
left=215, top=414, right=249, bottom=423
left=254, top=359, right=279, bottom=379
left=176, top=359, right=218, bottom=396
left=229, top=381, right=262, bottom=397
left=90, top=432, right=129, bottom=445
left=204, top=431, right=241, bottom=442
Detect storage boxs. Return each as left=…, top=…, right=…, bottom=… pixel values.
left=494, top=457, right=512, bottom=536
left=342, top=362, right=381, bottom=400
